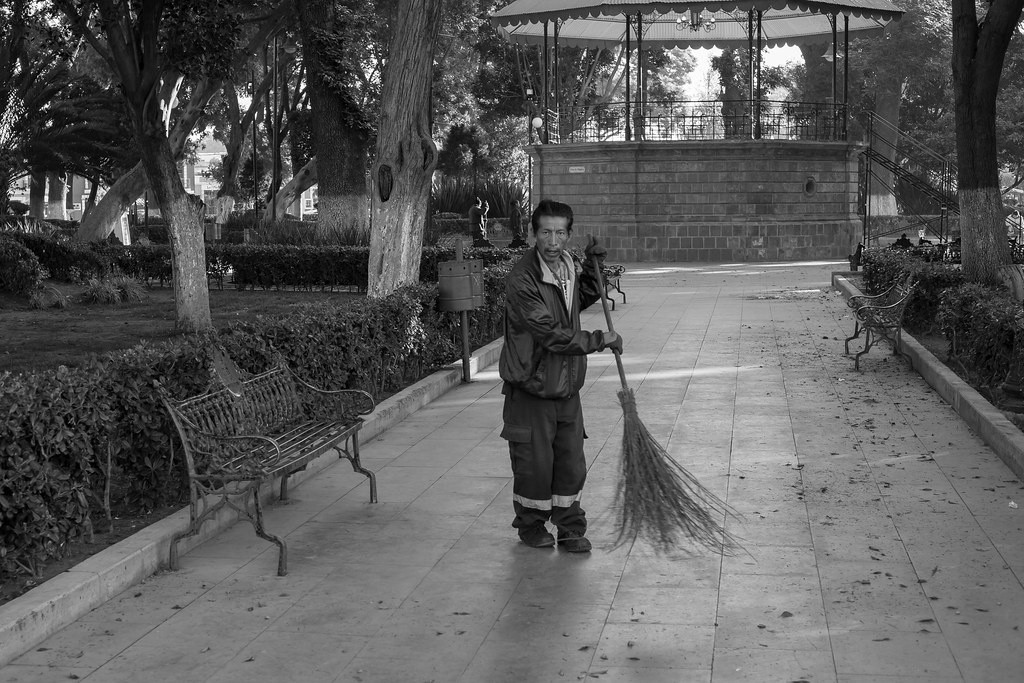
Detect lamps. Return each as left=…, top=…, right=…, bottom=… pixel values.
left=676, top=12, right=716, bottom=33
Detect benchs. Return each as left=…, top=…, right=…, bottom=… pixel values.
left=845, top=272, right=922, bottom=371
left=573, top=253, right=627, bottom=310
left=848, top=241, right=949, bottom=271
left=949, top=236, right=1018, bottom=262
left=148, top=358, right=374, bottom=579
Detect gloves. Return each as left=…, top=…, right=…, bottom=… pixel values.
left=604, top=331, right=623, bottom=355
left=585, top=237, right=607, bottom=265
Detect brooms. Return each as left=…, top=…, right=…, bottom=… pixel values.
left=587, top=234, right=764, bottom=566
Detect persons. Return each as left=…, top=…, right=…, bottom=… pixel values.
left=469, top=195, right=490, bottom=246
left=499, top=199, right=622, bottom=551
left=510, top=199, right=524, bottom=248
left=892, top=233, right=912, bottom=250
left=1005, top=210, right=1024, bottom=258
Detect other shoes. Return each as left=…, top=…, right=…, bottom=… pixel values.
left=557, top=533, right=592, bottom=552
left=518, top=526, right=555, bottom=547
